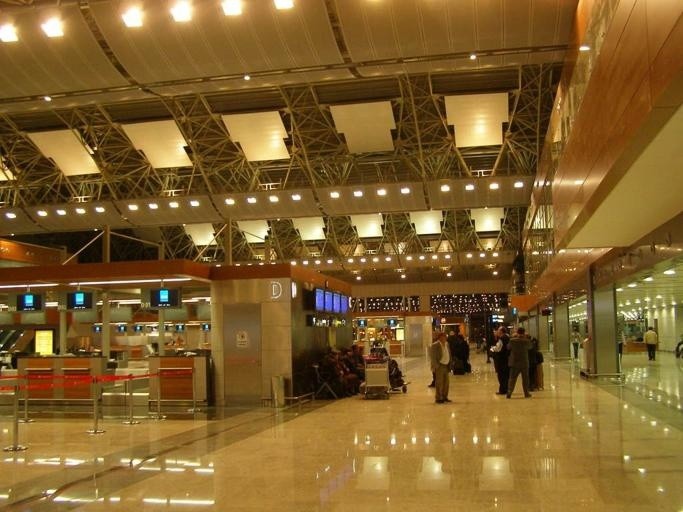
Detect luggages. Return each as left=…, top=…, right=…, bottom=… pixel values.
left=535, top=363, right=545, bottom=391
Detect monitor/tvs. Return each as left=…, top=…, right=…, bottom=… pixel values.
left=312, top=287, right=349, bottom=315
left=357, top=319, right=367, bottom=327
left=433, top=319, right=440, bottom=327
left=65, top=290, right=96, bottom=312
left=149, top=286, right=182, bottom=310
left=93, top=323, right=209, bottom=333
left=386, top=319, right=397, bottom=327
left=16, top=292, right=45, bottom=313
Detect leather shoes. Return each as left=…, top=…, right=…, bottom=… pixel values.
left=435, top=399, right=452, bottom=404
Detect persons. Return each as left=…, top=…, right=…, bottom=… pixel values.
left=429, top=332, right=454, bottom=405
left=643, top=326, right=658, bottom=361
left=569, top=326, right=583, bottom=359
left=426, top=336, right=439, bottom=388
left=616, top=323, right=627, bottom=360
left=445, top=325, right=543, bottom=400
left=316, top=344, right=368, bottom=399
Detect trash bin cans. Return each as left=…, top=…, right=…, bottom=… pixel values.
left=271, top=375, right=285, bottom=408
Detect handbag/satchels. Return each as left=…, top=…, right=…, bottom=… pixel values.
left=536, top=350, right=544, bottom=363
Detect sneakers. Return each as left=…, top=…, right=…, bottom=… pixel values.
left=495, top=391, right=533, bottom=399
left=427, top=381, right=435, bottom=389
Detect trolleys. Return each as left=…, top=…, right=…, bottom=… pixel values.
left=364, top=358, right=390, bottom=399
